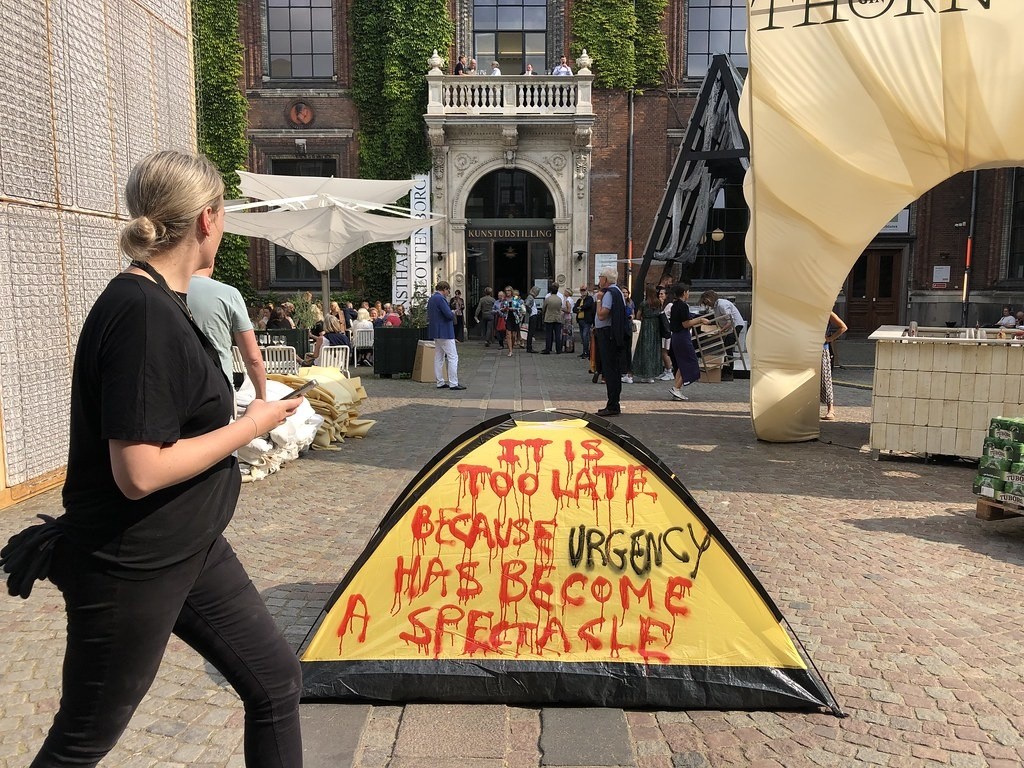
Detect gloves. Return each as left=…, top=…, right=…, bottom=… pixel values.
left=0, top=514, right=67, bottom=599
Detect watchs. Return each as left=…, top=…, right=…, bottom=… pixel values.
left=597, top=300, right=601, bottom=303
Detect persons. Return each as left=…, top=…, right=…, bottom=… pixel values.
left=819, top=311, right=848, bottom=420
left=29, top=150, right=304, bottom=768
left=450, top=290, right=465, bottom=342
left=594, top=267, right=626, bottom=416
left=589, top=289, right=745, bottom=403
left=995, top=307, right=1024, bottom=329
left=247, top=292, right=409, bottom=367
left=187, top=255, right=268, bottom=402
left=474, top=281, right=597, bottom=358
left=428, top=281, right=467, bottom=390
left=455, top=55, right=574, bottom=76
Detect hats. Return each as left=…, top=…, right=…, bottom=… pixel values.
left=580, top=285, right=587, bottom=290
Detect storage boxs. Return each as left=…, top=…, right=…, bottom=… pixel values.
left=411, top=340, right=447, bottom=382
left=973, top=416, right=1024, bottom=507
left=699, top=356, right=722, bottom=383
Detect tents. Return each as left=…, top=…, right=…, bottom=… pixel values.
left=293, top=407, right=848, bottom=719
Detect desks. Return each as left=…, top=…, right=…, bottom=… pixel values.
left=259, top=344, right=289, bottom=369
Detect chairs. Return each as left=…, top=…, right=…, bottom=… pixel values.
left=354, top=329, right=375, bottom=368
left=322, top=345, right=350, bottom=379
left=265, top=346, right=299, bottom=377
left=231, top=346, right=247, bottom=373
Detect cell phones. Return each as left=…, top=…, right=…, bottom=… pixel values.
left=281, top=380, right=320, bottom=401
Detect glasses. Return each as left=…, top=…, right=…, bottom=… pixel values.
left=505, top=290, right=511, bottom=291
left=1018, top=318, right=1022, bottom=320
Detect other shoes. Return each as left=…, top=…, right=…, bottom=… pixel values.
left=363, top=359, right=373, bottom=366
left=498, top=346, right=504, bottom=350
left=825, top=411, right=835, bottom=420
left=485, top=341, right=490, bottom=347
left=540, top=351, right=550, bottom=354
left=589, top=370, right=595, bottom=374
left=592, top=371, right=599, bottom=383
left=582, top=355, right=586, bottom=359
left=721, top=374, right=733, bottom=381
left=507, top=351, right=514, bottom=357
left=577, top=353, right=583, bottom=357
left=526, top=349, right=539, bottom=353
left=450, top=383, right=467, bottom=390
left=542, top=349, right=552, bottom=351
left=437, top=384, right=449, bottom=388
left=592, top=409, right=621, bottom=417
left=601, top=380, right=606, bottom=383
left=357, top=361, right=363, bottom=365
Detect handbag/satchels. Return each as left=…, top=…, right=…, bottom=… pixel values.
left=496, top=316, right=506, bottom=331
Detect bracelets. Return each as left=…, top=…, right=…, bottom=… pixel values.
left=241, top=416, right=257, bottom=439
left=698, top=317, right=703, bottom=325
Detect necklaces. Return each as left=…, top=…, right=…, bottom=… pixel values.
left=148, top=262, right=193, bottom=320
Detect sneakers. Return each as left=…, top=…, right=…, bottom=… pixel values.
left=670, top=396, right=681, bottom=400
left=641, top=378, right=654, bottom=383
left=622, top=374, right=633, bottom=384
left=668, top=386, right=689, bottom=400
left=655, top=373, right=675, bottom=381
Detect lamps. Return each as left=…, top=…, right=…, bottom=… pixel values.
left=545, top=70, right=551, bottom=75
left=574, top=251, right=587, bottom=260
left=503, top=246, right=517, bottom=258
left=696, top=233, right=706, bottom=243
left=434, top=252, right=447, bottom=260
left=712, top=188, right=725, bottom=241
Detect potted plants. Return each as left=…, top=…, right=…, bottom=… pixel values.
left=374, top=284, right=434, bottom=379
